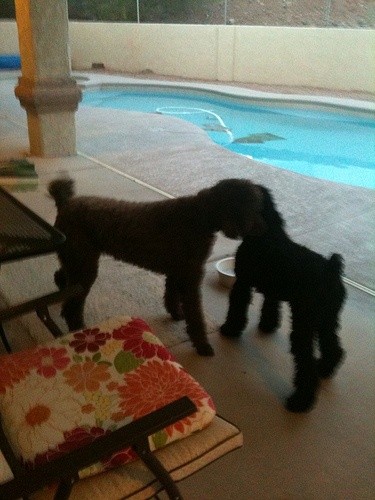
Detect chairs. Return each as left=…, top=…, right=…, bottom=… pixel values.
left=0, top=183, right=243, bottom=500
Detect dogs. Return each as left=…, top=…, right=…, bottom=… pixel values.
left=217, top=182, right=347, bottom=413
left=47, top=178, right=238, bottom=358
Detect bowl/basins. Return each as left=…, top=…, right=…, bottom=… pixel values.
left=217, top=257, right=236, bottom=287
